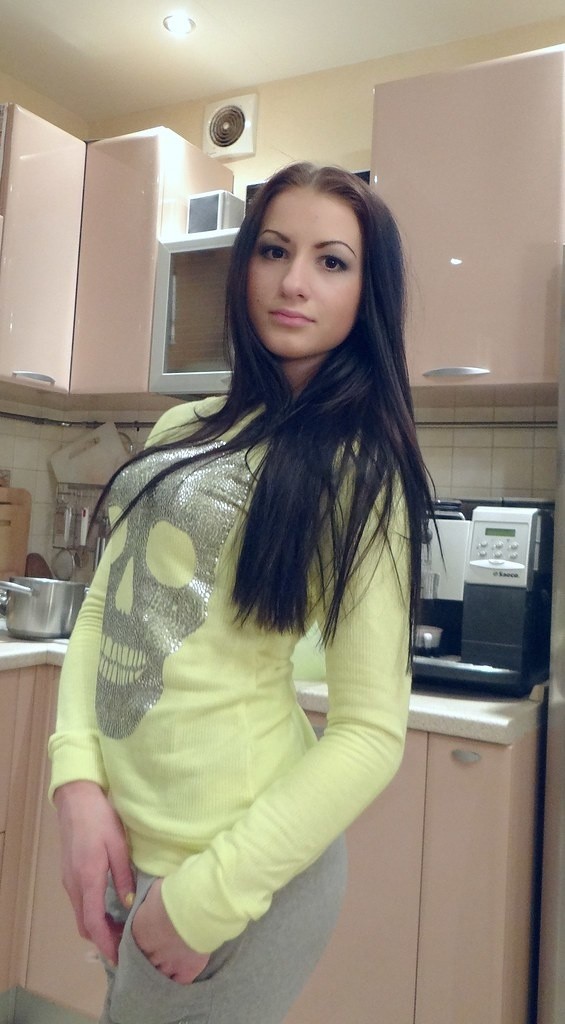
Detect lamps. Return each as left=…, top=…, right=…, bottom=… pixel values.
left=163, top=15, right=196, bottom=36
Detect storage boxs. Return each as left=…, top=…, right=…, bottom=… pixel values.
left=147, top=227, right=239, bottom=404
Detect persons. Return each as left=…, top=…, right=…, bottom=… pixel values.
left=45, top=162, right=446, bottom=1023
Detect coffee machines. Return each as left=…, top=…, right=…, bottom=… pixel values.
left=401, top=485, right=554, bottom=696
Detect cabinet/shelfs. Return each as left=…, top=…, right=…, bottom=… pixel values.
left=68, top=126, right=235, bottom=419
left=0, top=621, right=547, bottom=1023
left=0, top=105, right=88, bottom=409
left=361, top=43, right=564, bottom=412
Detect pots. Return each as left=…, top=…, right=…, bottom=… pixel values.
left=0, top=575, right=91, bottom=640
